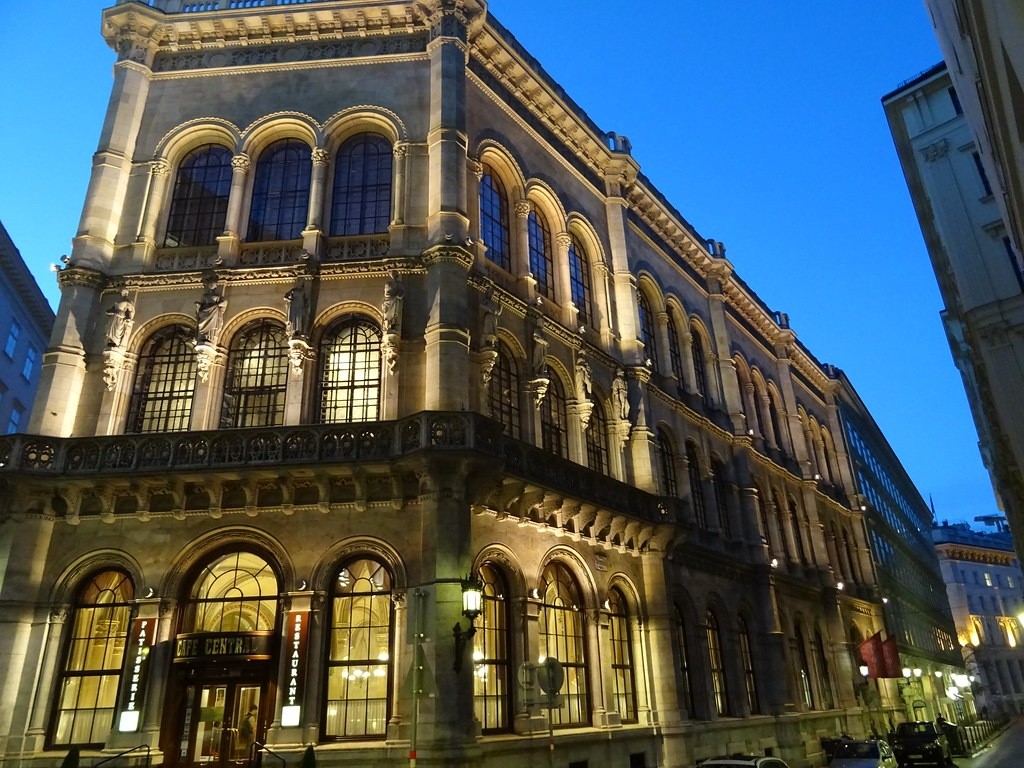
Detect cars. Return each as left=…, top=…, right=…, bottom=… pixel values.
left=828, top=740, right=898, bottom=768
left=696, top=755, right=789, bottom=768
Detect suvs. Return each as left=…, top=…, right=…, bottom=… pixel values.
left=893, top=722, right=953, bottom=768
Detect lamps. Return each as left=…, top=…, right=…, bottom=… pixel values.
left=454, top=574, right=483, bottom=648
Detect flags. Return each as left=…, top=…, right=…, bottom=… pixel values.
left=882, top=634, right=903, bottom=678
left=861, top=633, right=888, bottom=679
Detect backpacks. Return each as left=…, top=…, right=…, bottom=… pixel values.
left=240, top=711, right=255, bottom=739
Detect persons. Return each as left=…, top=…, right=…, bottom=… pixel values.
left=104, top=289, right=135, bottom=347
left=384, top=270, right=407, bottom=329
left=613, top=368, right=628, bottom=420
left=982, top=706, right=988, bottom=719
left=479, top=291, right=503, bottom=337
left=532, top=318, right=550, bottom=378
left=889, top=718, right=895, bottom=732
left=978, top=707, right=983, bottom=720
left=936, top=713, right=946, bottom=721
left=870, top=719, right=878, bottom=735
left=213, top=721, right=221, bottom=752
left=576, top=349, right=591, bottom=400
left=283, top=277, right=310, bottom=335
left=243, top=705, right=257, bottom=758
left=194, top=280, right=228, bottom=344
